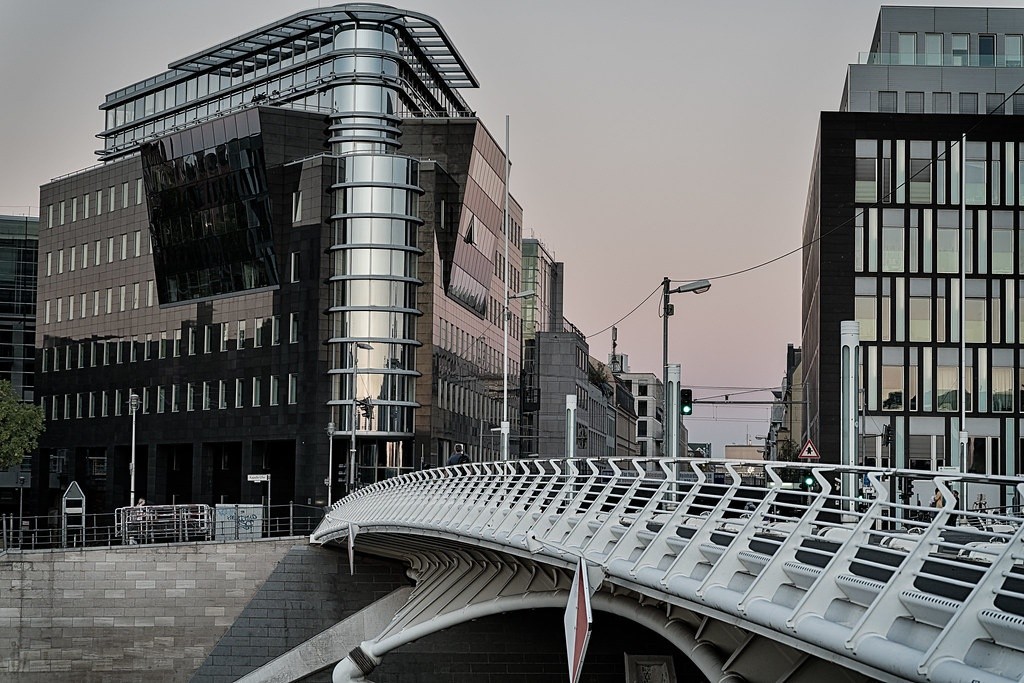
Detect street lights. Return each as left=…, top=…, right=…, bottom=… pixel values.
left=502, top=290, right=535, bottom=504
left=756, top=436, right=768, bottom=496
left=17, top=477, right=26, bottom=548
left=327, top=422, right=335, bottom=510
left=129, top=395, right=142, bottom=546
left=757, top=448, right=767, bottom=494
left=661, top=280, right=713, bottom=506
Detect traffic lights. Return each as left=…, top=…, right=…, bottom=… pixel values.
left=680, top=388, right=694, bottom=417
left=421, top=462, right=431, bottom=479
left=806, top=470, right=813, bottom=486
left=359, top=399, right=374, bottom=419
left=354, top=463, right=362, bottom=485
left=884, top=426, right=894, bottom=447
left=906, top=478, right=914, bottom=497
left=339, top=464, right=349, bottom=485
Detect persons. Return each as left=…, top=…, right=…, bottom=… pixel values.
left=446, top=444, right=470, bottom=467
left=129, top=498, right=147, bottom=545
left=928, top=487, right=961, bottom=512
